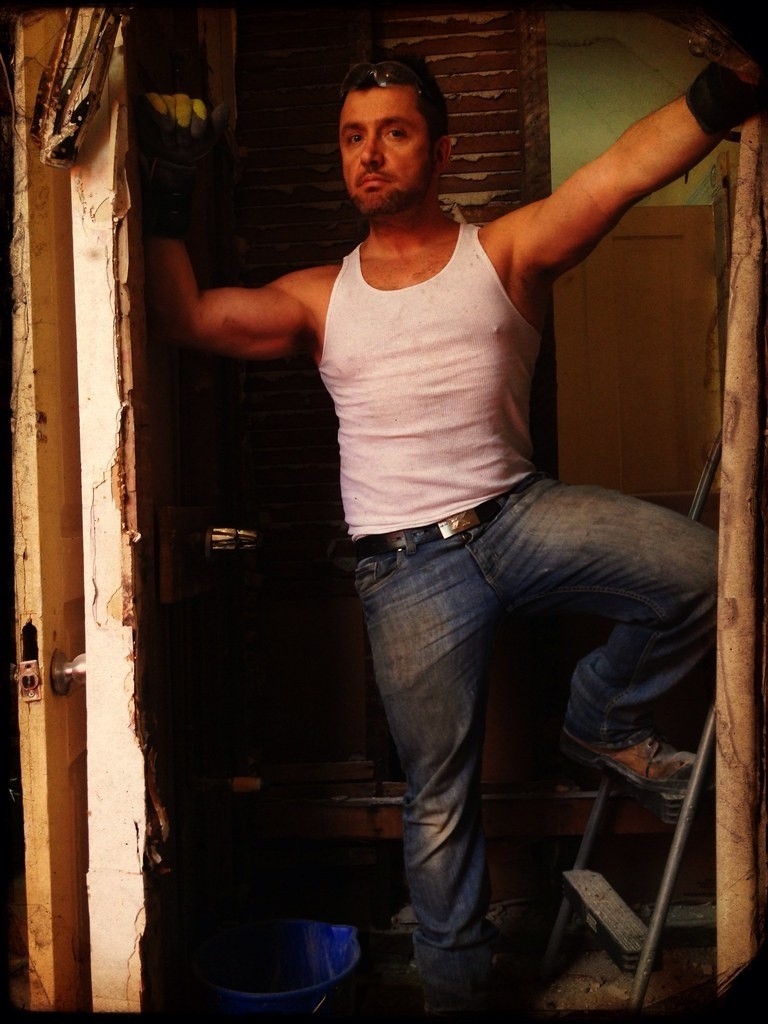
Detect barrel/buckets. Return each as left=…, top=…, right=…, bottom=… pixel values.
left=193, top=916, right=361, bottom=1016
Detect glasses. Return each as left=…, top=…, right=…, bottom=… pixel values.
left=338, top=62, right=443, bottom=116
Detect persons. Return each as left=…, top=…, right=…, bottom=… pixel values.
left=133, top=46, right=768, bottom=1013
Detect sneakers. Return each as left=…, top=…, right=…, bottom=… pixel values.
left=557, top=723, right=700, bottom=792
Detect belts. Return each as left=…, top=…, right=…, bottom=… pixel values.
left=356, top=496, right=508, bottom=562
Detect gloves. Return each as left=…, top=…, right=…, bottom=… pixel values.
left=685, top=53, right=767, bottom=135
left=136, top=92, right=230, bottom=239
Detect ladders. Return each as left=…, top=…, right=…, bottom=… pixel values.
left=540, top=428, right=726, bottom=1014
